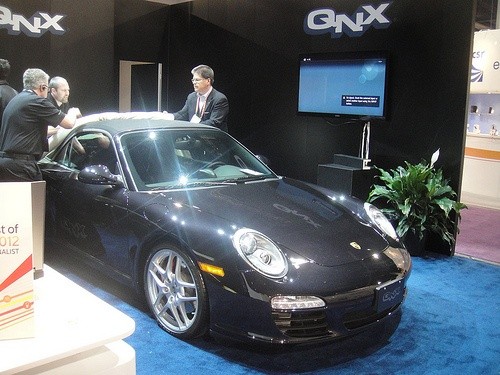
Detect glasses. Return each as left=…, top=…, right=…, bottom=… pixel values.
left=191, top=78, right=208, bottom=84
left=41, top=84, right=51, bottom=92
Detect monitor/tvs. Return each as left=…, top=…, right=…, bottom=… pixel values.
left=296, top=50, right=389, bottom=120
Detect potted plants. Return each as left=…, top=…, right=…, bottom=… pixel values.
left=367, top=147, right=469, bottom=257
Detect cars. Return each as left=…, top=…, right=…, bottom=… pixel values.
left=37, top=119, right=413, bottom=375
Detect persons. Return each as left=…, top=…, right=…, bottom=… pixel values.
left=0, top=67, right=82, bottom=183
left=46, top=76, right=71, bottom=137
left=162, top=64, right=231, bottom=164
left=0, top=59, right=18, bottom=125
left=71, top=132, right=117, bottom=174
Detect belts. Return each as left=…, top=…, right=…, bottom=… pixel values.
left=0, top=152, right=36, bottom=160
